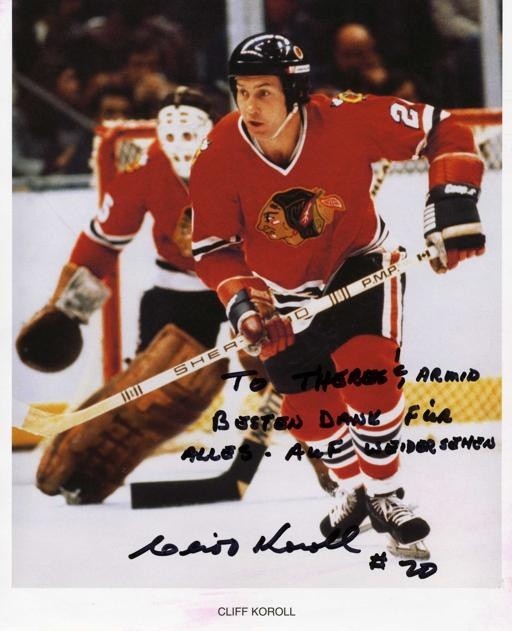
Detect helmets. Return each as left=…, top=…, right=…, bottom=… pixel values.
left=155, top=87, right=213, bottom=179
left=229, top=33, right=313, bottom=114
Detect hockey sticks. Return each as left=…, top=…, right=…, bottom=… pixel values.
left=131, top=158, right=393, bottom=509
left=12, top=246, right=440, bottom=438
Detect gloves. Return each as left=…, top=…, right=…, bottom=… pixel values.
left=422, top=150, right=488, bottom=272
left=213, top=276, right=293, bottom=360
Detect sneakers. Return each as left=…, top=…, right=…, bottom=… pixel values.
left=368, top=493, right=429, bottom=544
left=320, top=488, right=368, bottom=543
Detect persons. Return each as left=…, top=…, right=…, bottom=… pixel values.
left=256, top=186, right=348, bottom=248
left=14, top=0, right=503, bottom=198
left=188, top=31, right=485, bottom=545
left=16, top=85, right=343, bottom=508
